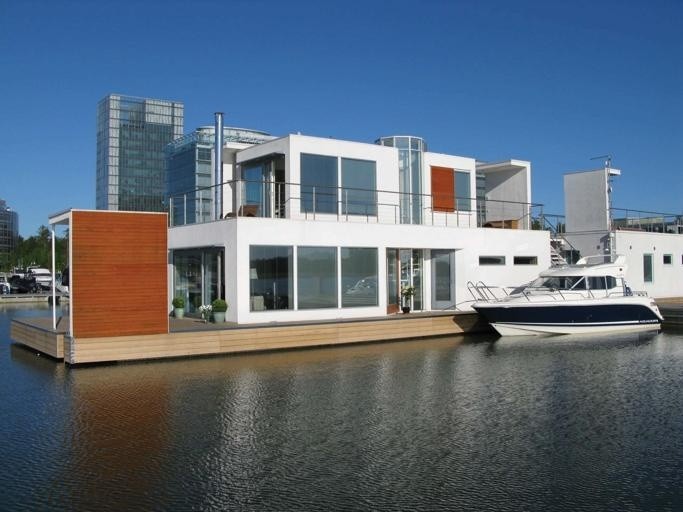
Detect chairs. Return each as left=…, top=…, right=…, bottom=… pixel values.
left=223, top=202, right=261, bottom=218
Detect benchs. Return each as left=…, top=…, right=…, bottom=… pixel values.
left=486, top=219, right=518, bottom=229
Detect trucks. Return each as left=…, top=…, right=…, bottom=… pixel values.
left=0, top=265, right=52, bottom=296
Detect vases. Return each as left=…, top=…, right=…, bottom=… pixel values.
left=401, top=307, right=412, bottom=313
left=203, top=313, right=209, bottom=327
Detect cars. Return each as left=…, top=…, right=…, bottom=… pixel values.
left=339, top=264, right=421, bottom=306
left=173, top=253, right=216, bottom=303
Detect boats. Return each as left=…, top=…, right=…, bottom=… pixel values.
left=466, top=252, right=662, bottom=335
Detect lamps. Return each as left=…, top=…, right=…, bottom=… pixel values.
left=249, top=267, right=258, bottom=310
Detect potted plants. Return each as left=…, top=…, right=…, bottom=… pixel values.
left=212, top=297, right=228, bottom=323
left=170, top=297, right=185, bottom=320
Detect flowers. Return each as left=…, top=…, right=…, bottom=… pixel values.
left=398, top=282, right=416, bottom=306
left=198, top=302, right=212, bottom=313
